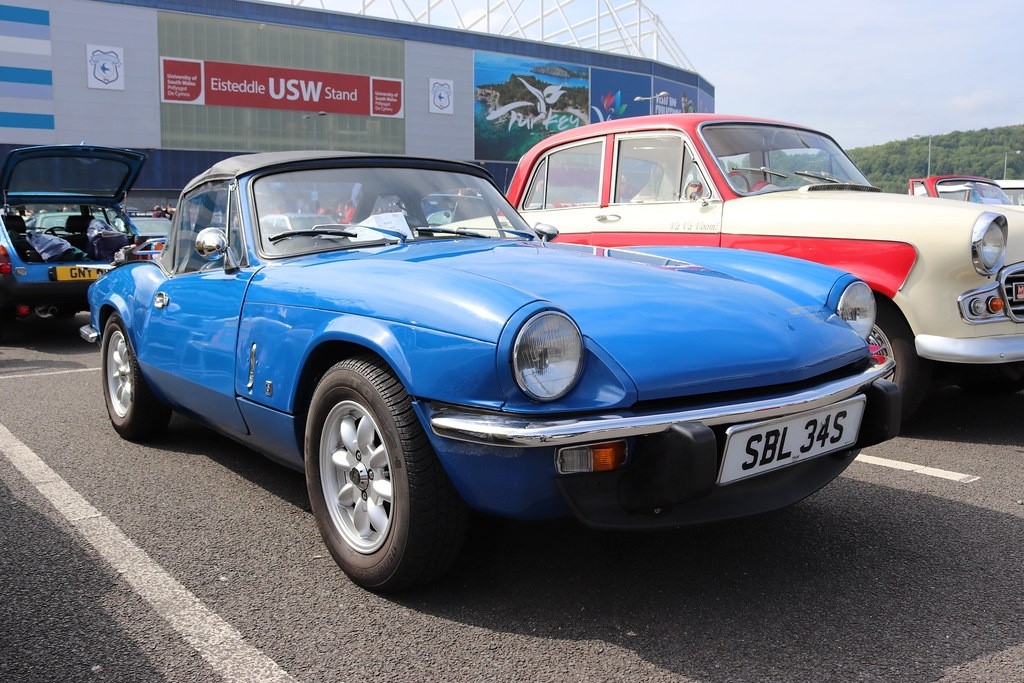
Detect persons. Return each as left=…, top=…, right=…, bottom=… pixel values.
left=680, top=92, right=688, bottom=113
left=15, top=205, right=70, bottom=216
left=153, top=203, right=175, bottom=220
left=318, top=199, right=356, bottom=224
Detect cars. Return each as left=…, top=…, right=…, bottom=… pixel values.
left=79, top=149, right=901, bottom=596
left=25, top=213, right=127, bottom=243
left=257, top=214, right=338, bottom=233
left=114, top=216, right=172, bottom=250
left=907, top=172, right=1024, bottom=207
left=1, top=140, right=154, bottom=334
left=431, top=111, right=1024, bottom=427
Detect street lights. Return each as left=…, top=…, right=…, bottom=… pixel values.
left=914, top=132, right=951, bottom=178
left=1003, top=150, right=1022, bottom=180
left=634, top=91, right=670, bottom=115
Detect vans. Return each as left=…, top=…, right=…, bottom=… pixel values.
left=420, top=193, right=501, bottom=228
